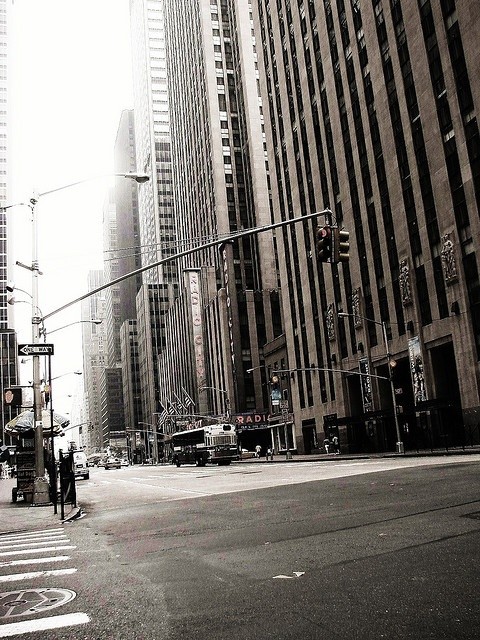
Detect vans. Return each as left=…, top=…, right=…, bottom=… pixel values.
left=68, top=452, right=90, bottom=479
left=88, top=461, right=95, bottom=468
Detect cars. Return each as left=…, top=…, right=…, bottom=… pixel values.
left=106, top=458, right=121, bottom=469
left=97, top=461, right=106, bottom=467
left=121, top=458, right=129, bottom=467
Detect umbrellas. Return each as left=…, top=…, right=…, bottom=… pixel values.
left=4, top=411, right=63, bottom=437
left=47, top=411, right=70, bottom=428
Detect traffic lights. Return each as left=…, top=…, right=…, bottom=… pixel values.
left=316, top=224, right=335, bottom=265
left=333, top=226, right=350, bottom=267
left=4, top=386, right=28, bottom=410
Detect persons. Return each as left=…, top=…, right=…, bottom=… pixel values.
left=323, top=438, right=331, bottom=454
left=332, top=434, right=340, bottom=453
left=255, top=444, right=262, bottom=458
left=265, top=445, right=273, bottom=460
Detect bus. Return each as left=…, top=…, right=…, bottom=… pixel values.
left=170, top=423, right=240, bottom=467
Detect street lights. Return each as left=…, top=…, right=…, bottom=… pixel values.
left=265, top=365, right=389, bottom=390
left=44, top=317, right=107, bottom=410
left=335, top=309, right=413, bottom=456
left=45, top=370, right=84, bottom=382
left=23, top=169, right=151, bottom=505
